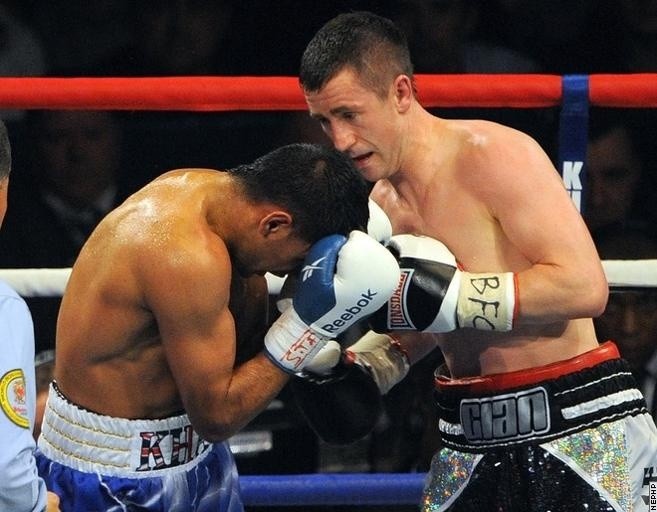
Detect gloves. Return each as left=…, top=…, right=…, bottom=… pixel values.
left=262, top=234, right=514, bottom=377
left=296, top=330, right=412, bottom=446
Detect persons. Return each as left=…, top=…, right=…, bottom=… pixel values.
left=0, top=10, right=657, bottom=512
